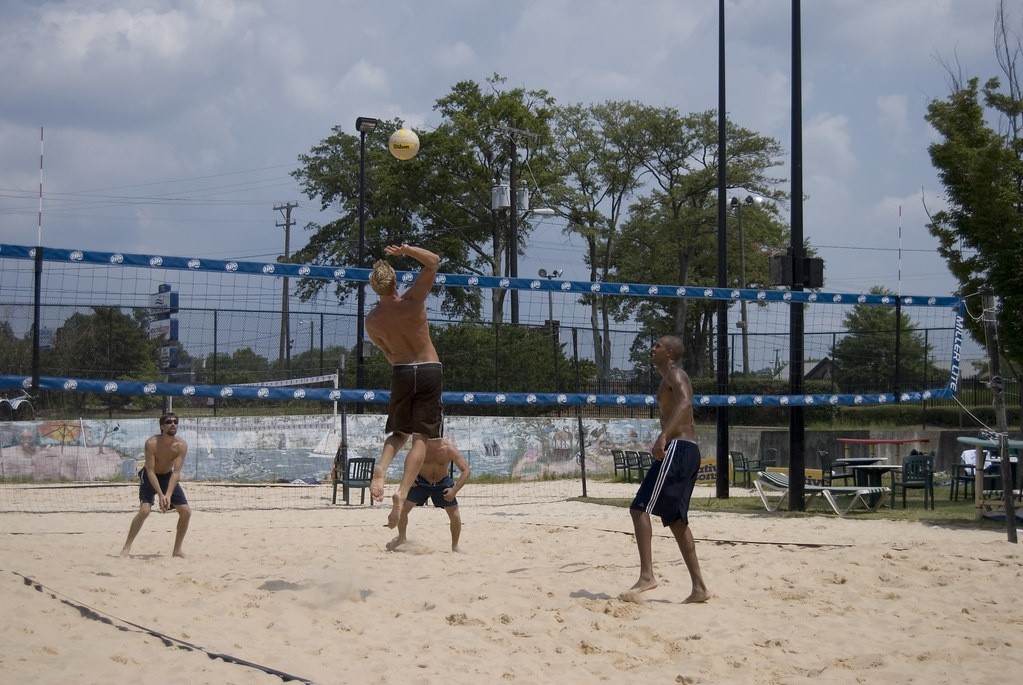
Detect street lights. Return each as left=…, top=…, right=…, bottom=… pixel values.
left=353, top=116, right=380, bottom=414
left=727, top=195, right=764, bottom=376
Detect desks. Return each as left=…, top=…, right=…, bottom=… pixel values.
left=836, top=457, right=888, bottom=486
left=848, top=465, right=902, bottom=508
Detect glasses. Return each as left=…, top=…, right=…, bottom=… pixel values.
left=162, top=420, right=178, bottom=425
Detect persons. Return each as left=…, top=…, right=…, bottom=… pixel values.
left=364, top=244, right=444, bottom=530
left=629, top=335, right=711, bottom=605
left=385, top=436, right=472, bottom=557
left=118, top=413, right=191, bottom=560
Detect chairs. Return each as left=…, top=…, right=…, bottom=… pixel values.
left=949, top=464, right=976, bottom=501
left=333, top=458, right=376, bottom=506
left=753, top=472, right=891, bottom=515
left=818, top=451, right=855, bottom=487
left=611, top=450, right=655, bottom=484
left=760, top=447, right=778, bottom=471
left=890, top=451, right=936, bottom=510
left=730, top=451, right=762, bottom=488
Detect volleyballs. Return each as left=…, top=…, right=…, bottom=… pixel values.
left=389, top=128, right=420, bottom=161
